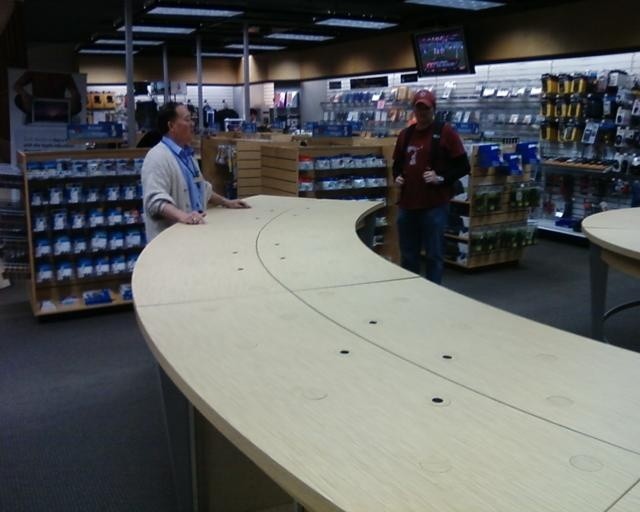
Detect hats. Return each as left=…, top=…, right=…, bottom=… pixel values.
left=412, top=89, right=436, bottom=108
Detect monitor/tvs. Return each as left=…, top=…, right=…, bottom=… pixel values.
left=411, top=24, right=475, bottom=77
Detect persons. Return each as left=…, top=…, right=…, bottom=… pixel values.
left=419, top=35, right=466, bottom=72
left=12, top=69, right=85, bottom=122
left=141, top=100, right=252, bottom=242
left=392, top=87, right=473, bottom=284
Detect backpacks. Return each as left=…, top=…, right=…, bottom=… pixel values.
left=449, top=180, right=464, bottom=200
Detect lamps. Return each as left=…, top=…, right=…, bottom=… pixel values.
left=79, top=49, right=138, bottom=54
left=225, top=43, right=286, bottom=51
left=195, top=51, right=245, bottom=58
left=118, top=24, right=195, bottom=34
left=93, top=38, right=163, bottom=45
left=147, top=6, right=243, bottom=17
left=315, top=16, right=398, bottom=30
left=264, top=32, right=334, bottom=43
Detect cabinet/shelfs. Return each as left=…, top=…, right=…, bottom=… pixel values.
left=215, top=115, right=538, bottom=272
left=4, top=143, right=149, bottom=319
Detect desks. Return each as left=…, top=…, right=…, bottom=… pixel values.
left=581, top=207, right=640, bottom=352
left=130, top=194, right=640, bottom=512
